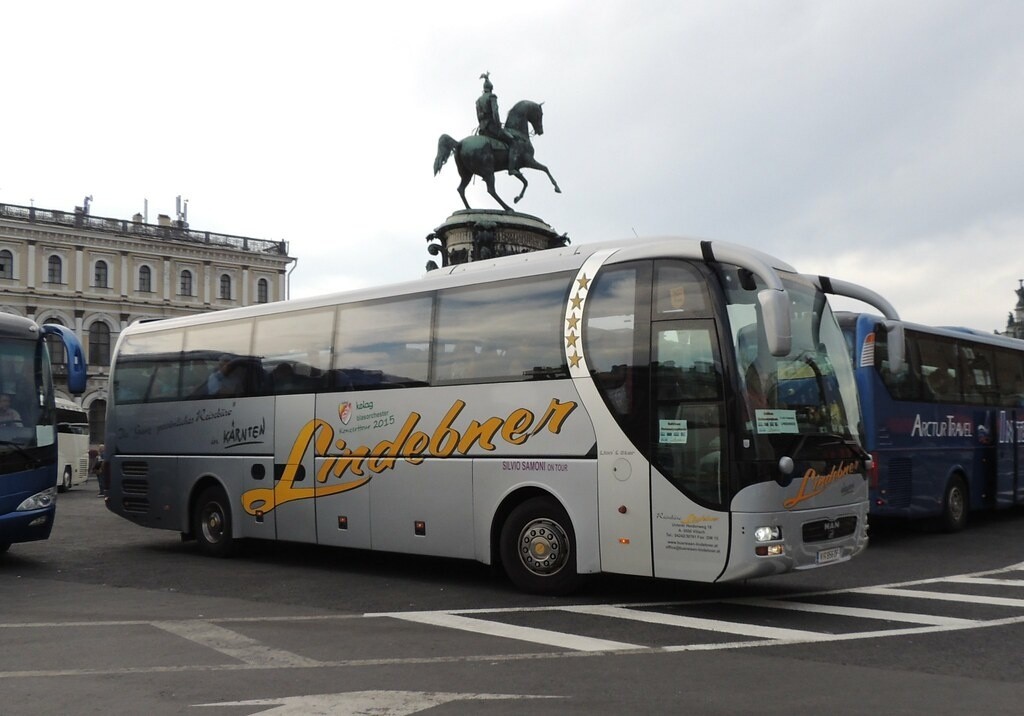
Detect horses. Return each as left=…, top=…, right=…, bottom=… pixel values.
left=433, top=100, right=562, bottom=212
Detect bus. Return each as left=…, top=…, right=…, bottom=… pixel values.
left=0, top=311, right=88, bottom=556
left=39, top=391, right=90, bottom=493
left=735, top=308, right=1024, bottom=535
left=100, top=238, right=907, bottom=599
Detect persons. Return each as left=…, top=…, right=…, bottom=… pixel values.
left=0, top=393, right=24, bottom=427
left=150, top=354, right=274, bottom=399
left=476, top=72, right=522, bottom=176
left=87, top=445, right=106, bottom=497
left=592, top=334, right=630, bottom=421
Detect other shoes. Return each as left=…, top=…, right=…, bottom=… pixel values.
left=97, top=493, right=104, bottom=498
